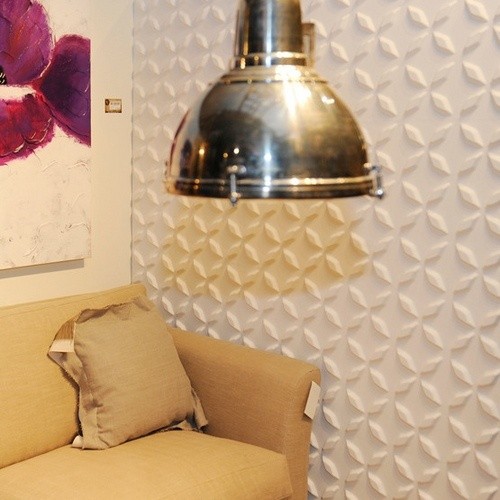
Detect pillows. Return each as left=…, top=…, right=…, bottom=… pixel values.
left=46, top=297, right=208, bottom=449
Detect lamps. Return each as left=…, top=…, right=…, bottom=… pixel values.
left=163, top=0, right=385, bottom=205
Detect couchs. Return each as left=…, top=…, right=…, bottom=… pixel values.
left=0, top=281, right=323, bottom=498
